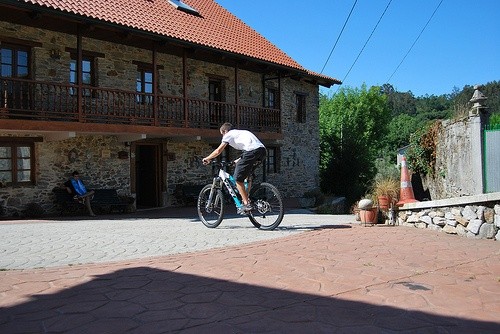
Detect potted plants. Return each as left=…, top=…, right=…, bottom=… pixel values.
left=365, top=176, right=401, bottom=209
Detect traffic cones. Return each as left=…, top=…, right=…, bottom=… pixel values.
left=395, top=155, right=419, bottom=205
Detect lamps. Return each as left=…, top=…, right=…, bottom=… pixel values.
left=49, top=49, right=60, bottom=59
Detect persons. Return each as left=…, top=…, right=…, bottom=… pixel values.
left=59, top=171, right=97, bottom=217
left=202, top=123, right=267, bottom=214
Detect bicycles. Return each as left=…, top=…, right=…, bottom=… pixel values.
left=197, top=159, right=285, bottom=232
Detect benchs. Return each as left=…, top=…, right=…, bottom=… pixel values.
left=52, top=188, right=129, bottom=217
left=179, top=185, right=205, bottom=207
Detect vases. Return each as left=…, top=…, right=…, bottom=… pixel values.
left=360, top=207, right=378, bottom=223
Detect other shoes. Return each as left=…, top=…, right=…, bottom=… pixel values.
left=89, top=213, right=96, bottom=216
left=78, top=197, right=83, bottom=201
left=237, top=204, right=253, bottom=214
